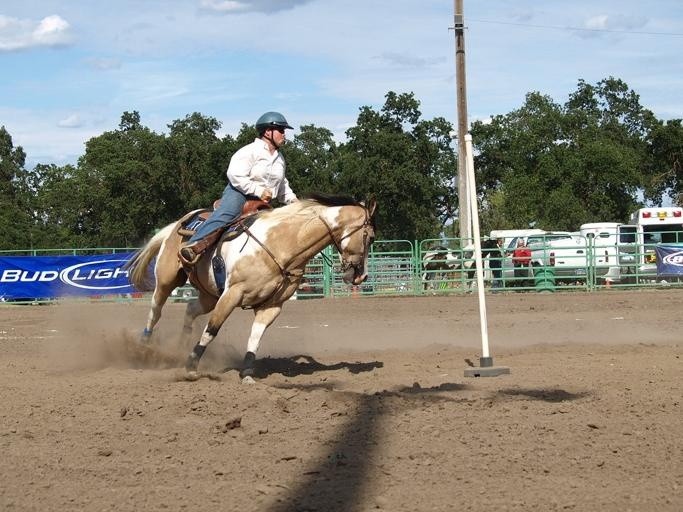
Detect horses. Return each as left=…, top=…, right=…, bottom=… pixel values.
left=420, top=240, right=501, bottom=291
left=116, top=190, right=380, bottom=385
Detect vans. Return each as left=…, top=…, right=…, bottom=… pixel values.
left=614, top=206, right=683, bottom=284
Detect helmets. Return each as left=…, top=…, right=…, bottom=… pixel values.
left=255, top=111, right=294, bottom=130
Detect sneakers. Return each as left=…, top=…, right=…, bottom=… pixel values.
left=180, top=247, right=197, bottom=267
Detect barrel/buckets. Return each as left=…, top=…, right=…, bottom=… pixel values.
left=533, top=264, right=556, bottom=294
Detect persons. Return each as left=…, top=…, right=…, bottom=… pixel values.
left=511, top=239, right=531, bottom=292
left=176, top=109, right=300, bottom=263
left=489, top=238, right=510, bottom=294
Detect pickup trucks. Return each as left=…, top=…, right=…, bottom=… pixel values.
left=498, top=232, right=610, bottom=286
